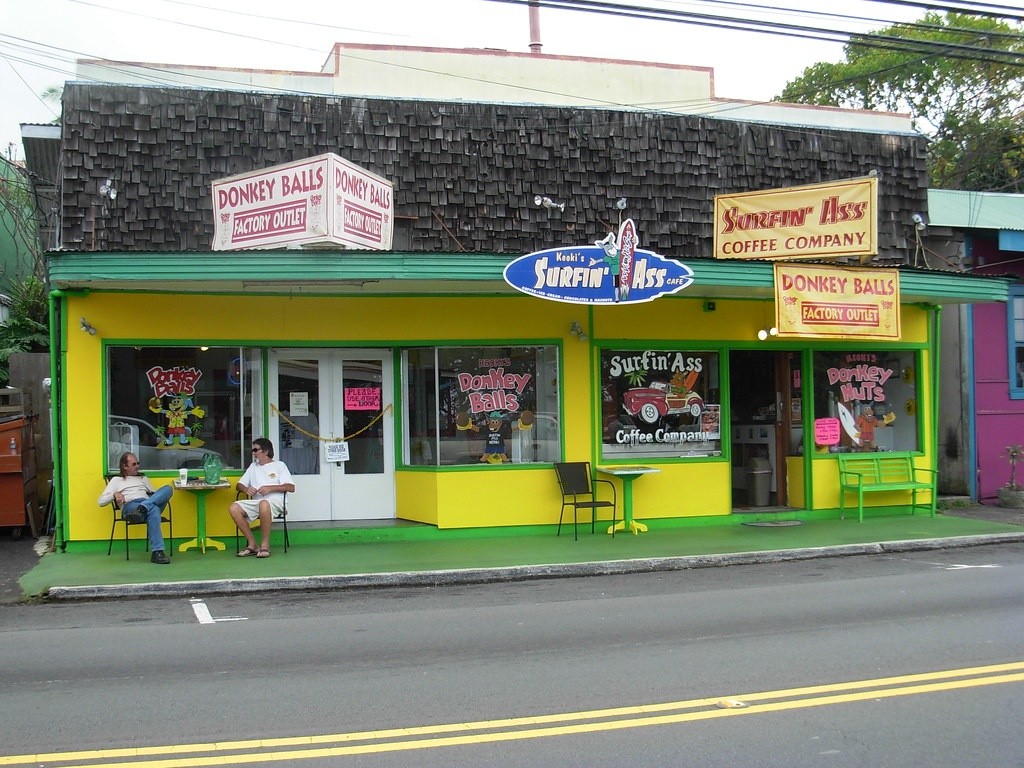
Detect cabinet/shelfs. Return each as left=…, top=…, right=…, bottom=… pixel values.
left=535, top=346, right=560, bottom=461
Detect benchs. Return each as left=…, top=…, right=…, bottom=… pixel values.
left=838, top=450, right=938, bottom=523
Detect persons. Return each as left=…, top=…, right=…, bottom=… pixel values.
left=228, top=438, right=295, bottom=559
left=97, top=452, right=173, bottom=564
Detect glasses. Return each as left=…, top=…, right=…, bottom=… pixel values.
left=252, top=448, right=262, bottom=452
left=132, top=462, right=139, bottom=466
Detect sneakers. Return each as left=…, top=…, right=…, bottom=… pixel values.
left=151, top=550, right=170, bottom=563
left=126, top=505, right=149, bottom=523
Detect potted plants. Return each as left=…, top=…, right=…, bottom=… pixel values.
left=995, top=444, right=1023, bottom=509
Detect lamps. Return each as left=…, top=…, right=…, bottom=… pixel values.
left=533, top=195, right=564, bottom=213
left=99, top=176, right=116, bottom=200
left=569, top=321, right=587, bottom=343
left=912, top=214, right=925, bottom=231
left=79, top=316, right=95, bottom=335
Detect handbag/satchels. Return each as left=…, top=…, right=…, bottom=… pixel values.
left=202, top=453, right=224, bottom=485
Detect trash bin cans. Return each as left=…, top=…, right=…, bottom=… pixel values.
left=745, top=457, right=772, bottom=506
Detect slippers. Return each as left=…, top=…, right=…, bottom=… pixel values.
left=236, top=547, right=256, bottom=556
left=256, top=549, right=272, bottom=558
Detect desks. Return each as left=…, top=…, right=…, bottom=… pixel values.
left=172, top=477, right=231, bottom=555
left=595, top=466, right=661, bottom=536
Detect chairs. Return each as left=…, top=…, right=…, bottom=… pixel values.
left=553, top=462, right=616, bottom=541
left=235, top=486, right=289, bottom=555
left=103, top=473, right=175, bottom=560
